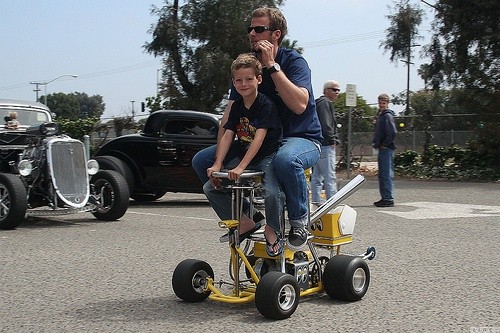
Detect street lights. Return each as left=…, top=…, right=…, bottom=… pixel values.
left=43, top=74, right=78, bottom=106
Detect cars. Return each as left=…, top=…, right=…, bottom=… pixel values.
left=90, top=108, right=224, bottom=202
left=0, top=97, right=130, bottom=231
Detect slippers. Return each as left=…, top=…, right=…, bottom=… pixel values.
left=263, top=230, right=282, bottom=257
left=231, top=222, right=261, bottom=247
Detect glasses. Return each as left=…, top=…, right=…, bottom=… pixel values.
left=247, top=26, right=276, bottom=34
left=326, top=88, right=340, bottom=92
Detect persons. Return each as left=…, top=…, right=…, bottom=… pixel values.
left=192, top=8, right=324, bottom=251
left=311, top=80, right=340, bottom=205
left=206, top=54, right=285, bottom=256
left=372, top=94, right=397, bottom=206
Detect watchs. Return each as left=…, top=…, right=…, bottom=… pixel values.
left=268, top=63, right=280, bottom=74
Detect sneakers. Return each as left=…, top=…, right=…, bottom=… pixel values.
left=219, top=211, right=266, bottom=242
left=286, top=226, right=308, bottom=250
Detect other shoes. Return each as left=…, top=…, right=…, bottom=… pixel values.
left=374, top=199, right=394, bottom=207
